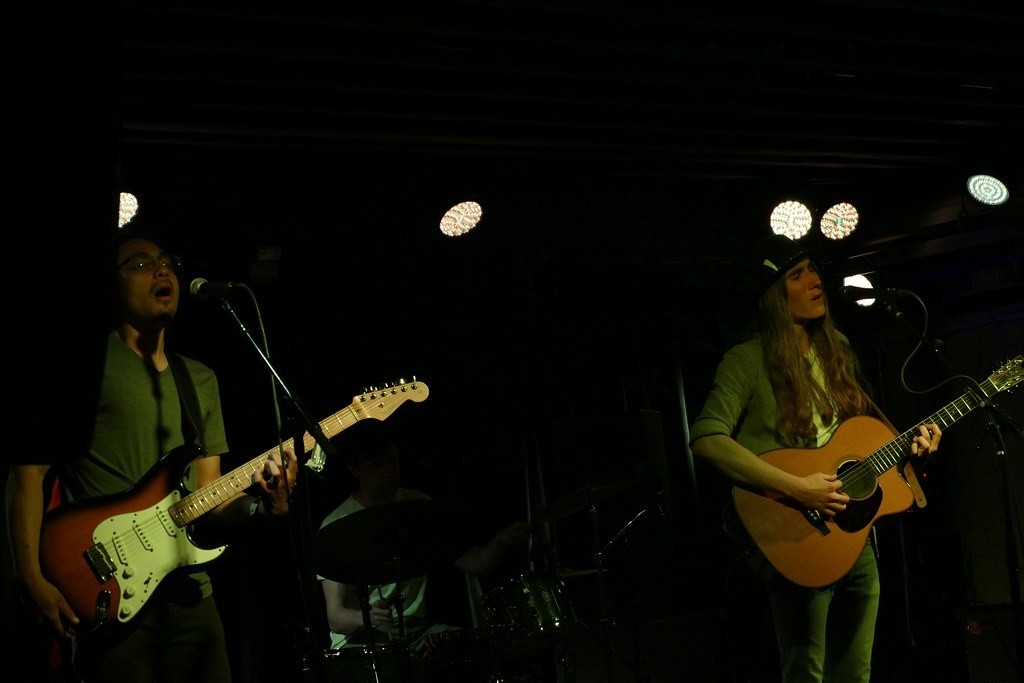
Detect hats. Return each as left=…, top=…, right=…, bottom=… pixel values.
left=744, top=234, right=810, bottom=305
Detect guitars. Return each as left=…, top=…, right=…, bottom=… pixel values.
left=733, top=352, right=1024, bottom=587
left=41, top=374, right=429, bottom=631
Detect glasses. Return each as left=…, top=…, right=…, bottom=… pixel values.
left=116, top=252, right=181, bottom=273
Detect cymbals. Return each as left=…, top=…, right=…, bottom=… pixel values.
left=311, top=498, right=474, bottom=586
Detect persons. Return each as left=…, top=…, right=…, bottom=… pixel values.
left=685, top=231, right=945, bottom=683
left=4, top=230, right=295, bottom=683
left=313, top=445, right=533, bottom=658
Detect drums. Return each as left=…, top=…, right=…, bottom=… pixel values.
left=317, top=644, right=411, bottom=682
left=476, top=568, right=577, bottom=660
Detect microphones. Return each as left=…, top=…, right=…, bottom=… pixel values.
left=839, top=286, right=913, bottom=303
left=189, top=278, right=246, bottom=300
